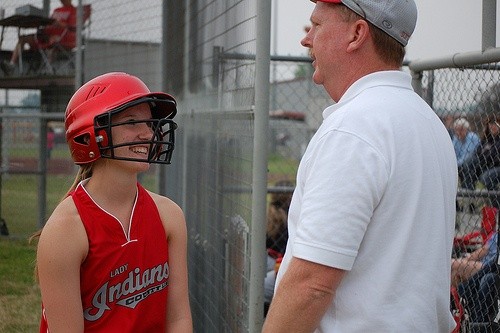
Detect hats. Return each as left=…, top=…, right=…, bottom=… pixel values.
left=310, top=0, right=417, bottom=47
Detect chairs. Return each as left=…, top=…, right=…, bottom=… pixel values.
left=34, top=4, right=91, bottom=76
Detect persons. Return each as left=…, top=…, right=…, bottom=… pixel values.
left=35, top=71, right=194, bottom=333
left=261, top=0, right=459, bottom=333
left=451, top=117, right=500, bottom=333
left=3, top=0, right=77, bottom=72
left=264, top=196, right=292, bottom=303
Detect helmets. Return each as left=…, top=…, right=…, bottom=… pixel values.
left=65, top=72, right=178, bottom=164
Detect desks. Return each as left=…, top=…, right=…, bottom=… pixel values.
left=0, top=14, right=56, bottom=73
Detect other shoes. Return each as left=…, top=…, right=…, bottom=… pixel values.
left=0, top=58, right=16, bottom=75
